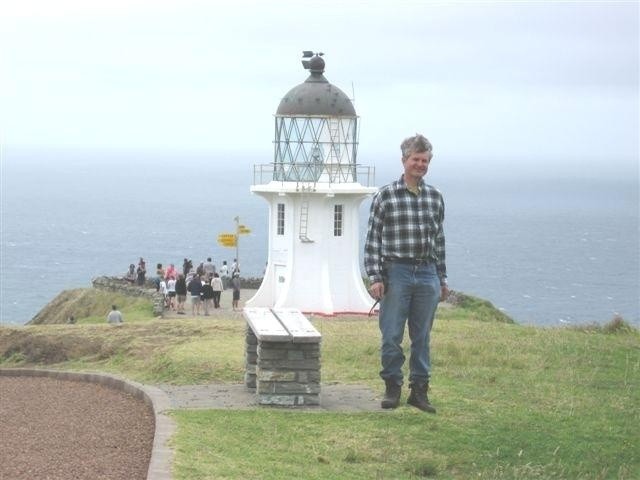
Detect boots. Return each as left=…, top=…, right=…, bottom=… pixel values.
left=407, top=383, right=436, bottom=412
left=381, top=378, right=401, bottom=408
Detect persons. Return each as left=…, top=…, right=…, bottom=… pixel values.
left=364, top=135, right=450, bottom=415
left=66, top=315, right=74, bottom=324
left=155, top=257, right=245, bottom=315
left=106, top=305, right=123, bottom=323
left=127, top=257, right=146, bottom=286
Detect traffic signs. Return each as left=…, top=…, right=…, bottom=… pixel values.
left=217, top=224, right=252, bottom=248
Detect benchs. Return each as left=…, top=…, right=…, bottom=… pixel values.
left=241, top=306, right=324, bottom=407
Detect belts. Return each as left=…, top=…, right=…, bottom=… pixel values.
left=385, top=256, right=432, bottom=264
